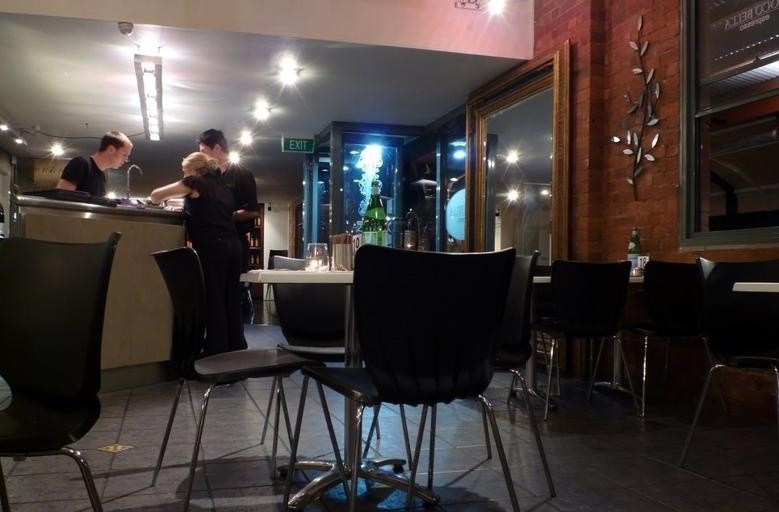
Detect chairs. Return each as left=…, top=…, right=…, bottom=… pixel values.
left=411, top=249, right=557, bottom=497
left=282, top=245, right=520, bottom=512
left=261, top=256, right=412, bottom=480
left=0, top=231, right=122, bottom=512
left=148, top=247, right=350, bottom=512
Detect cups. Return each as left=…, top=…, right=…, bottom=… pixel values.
left=304, top=243, right=330, bottom=272
left=330, top=232, right=352, bottom=270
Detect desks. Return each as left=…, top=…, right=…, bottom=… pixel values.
left=240, top=269, right=441, bottom=511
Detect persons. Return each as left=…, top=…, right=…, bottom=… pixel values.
left=53, top=130, right=131, bottom=199
left=149, top=151, right=239, bottom=353
left=198, top=128, right=259, bottom=349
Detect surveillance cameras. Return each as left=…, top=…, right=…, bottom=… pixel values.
left=118, top=22, right=134, bottom=37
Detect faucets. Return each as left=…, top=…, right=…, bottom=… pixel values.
left=127, top=164, right=143, bottom=198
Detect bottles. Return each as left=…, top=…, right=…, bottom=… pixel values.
left=627, top=226, right=641, bottom=267
left=250, top=230, right=260, bottom=263
left=362, top=179, right=388, bottom=247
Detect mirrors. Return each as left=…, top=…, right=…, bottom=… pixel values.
left=465, top=40, right=570, bottom=266
